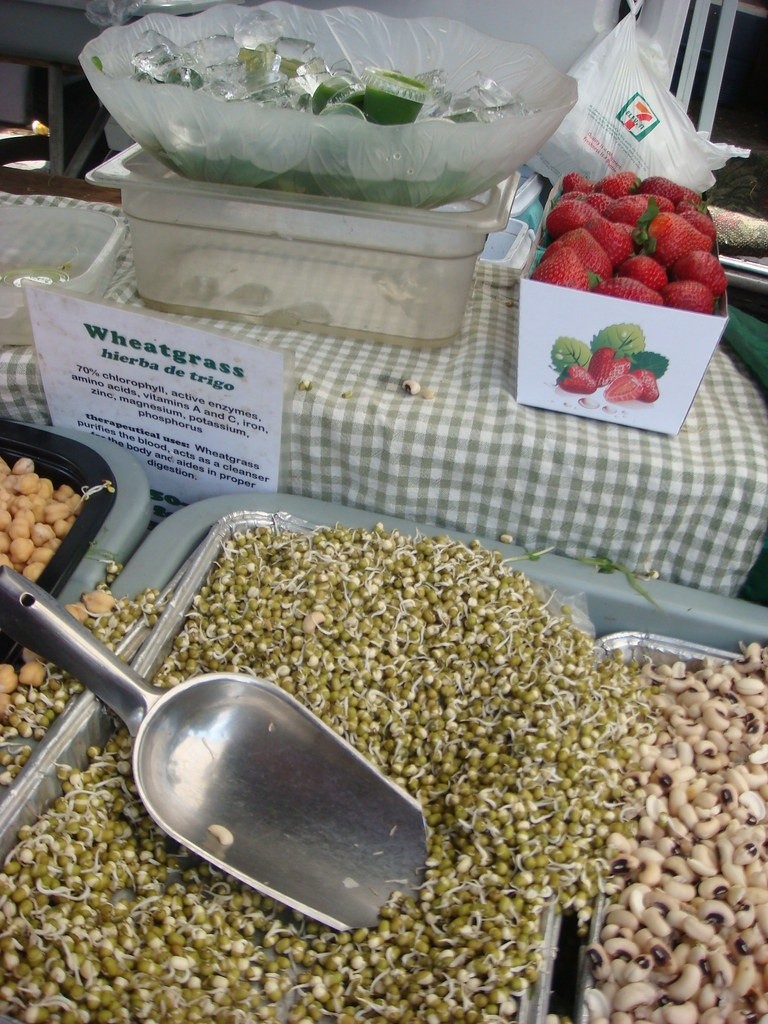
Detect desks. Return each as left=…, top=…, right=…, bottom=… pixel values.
left=0, top=190, right=768, bottom=597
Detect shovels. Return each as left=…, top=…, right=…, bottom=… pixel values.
left=0, top=563, right=427, bottom=934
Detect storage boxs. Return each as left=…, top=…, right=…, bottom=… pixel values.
left=85, top=140, right=522, bottom=349
left=518, top=176, right=730, bottom=436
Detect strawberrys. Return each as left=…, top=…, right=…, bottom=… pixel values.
left=532, top=170, right=729, bottom=317
left=555, top=347, right=659, bottom=403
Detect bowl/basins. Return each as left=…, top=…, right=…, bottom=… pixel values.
left=78, top=0, right=578, bottom=209
